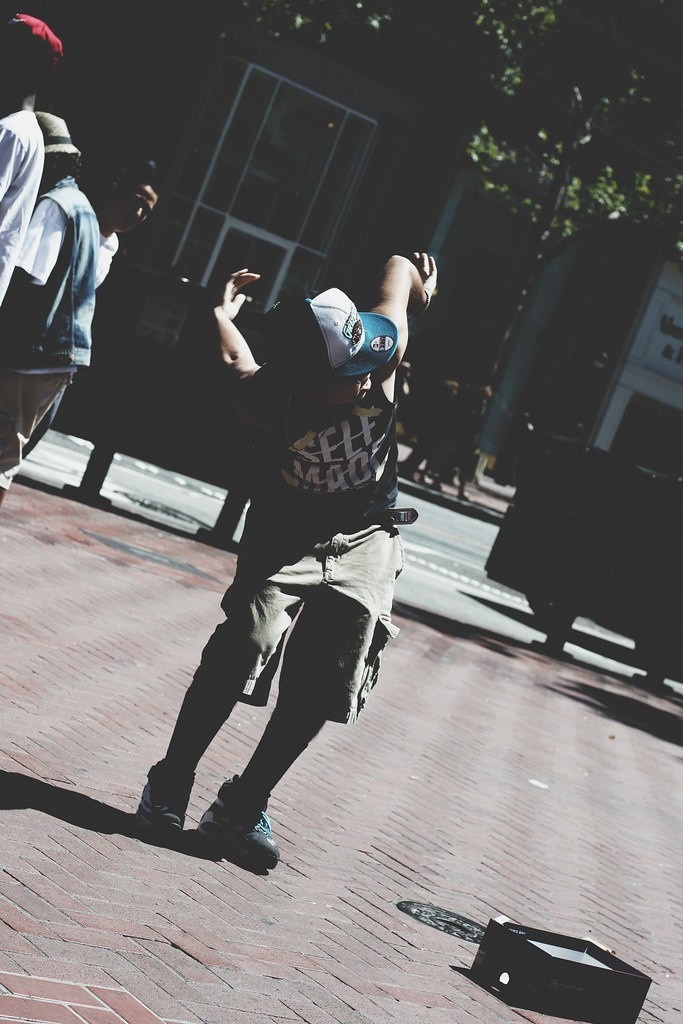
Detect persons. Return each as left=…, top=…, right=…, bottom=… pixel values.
left=0, top=13, right=64, bottom=310
left=22, top=167, right=158, bottom=462
left=0, top=110, right=100, bottom=516
left=135, top=251, right=438, bottom=870
left=397, top=361, right=493, bottom=501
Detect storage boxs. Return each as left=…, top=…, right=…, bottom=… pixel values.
left=469, top=918, right=653, bottom=1024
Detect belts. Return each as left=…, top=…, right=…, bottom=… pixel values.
left=355, top=507, right=419, bottom=525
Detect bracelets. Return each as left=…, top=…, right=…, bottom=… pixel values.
left=408, top=288, right=430, bottom=315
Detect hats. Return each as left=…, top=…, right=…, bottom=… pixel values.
left=35, top=112, right=81, bottom=159
left=1, top=13, right=64, bottom=70
left=264, top=286, right=399, bottom=377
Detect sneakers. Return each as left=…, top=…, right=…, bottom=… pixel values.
left=137, top=758, right=196, bottom=838
left=198, top=777, right=279, bottom=868
